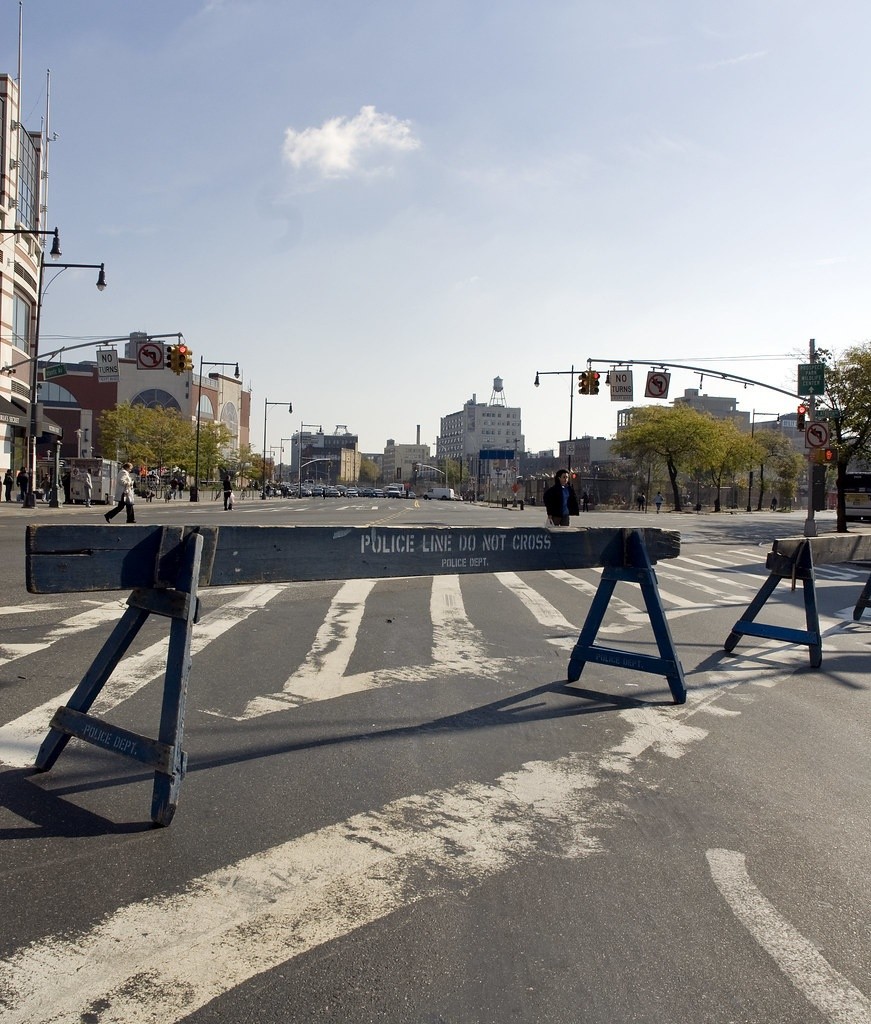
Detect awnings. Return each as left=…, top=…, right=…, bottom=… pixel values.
left=0, top=395, right=62, bottom=437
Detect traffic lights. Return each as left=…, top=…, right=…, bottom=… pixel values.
left=590, top=371, right=599, bottom=395
left=579, top=375, right=582, bottom=394
left=167, top=344, right=179, bottom=371
left=186, top=348, right=194, bottom=370
left=797, top=405, right=805, bottom=430
left=178, top=345, right=188, bottom=370
left=570, top=471, right=578, bottom=480
left=826, top=448, right=840, bottom=463
left=581, top=371, right=590, bottom=394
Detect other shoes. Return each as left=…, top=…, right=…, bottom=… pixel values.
left=104, top=513, right=110, bottom=523
left=126, top=520, right=136, bottom=523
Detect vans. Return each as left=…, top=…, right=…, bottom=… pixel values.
left=423, top=487, right=454, bottom=501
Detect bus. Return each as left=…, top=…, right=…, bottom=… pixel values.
left=836, top=471, right=871, bottom=523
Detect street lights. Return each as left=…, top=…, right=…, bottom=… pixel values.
left=279, top=436, right=298, bottom=483
left=262, top=397, right=293, bottom=500
left=269, top=445, right=284, bottom=485
left=298, top=422, right=324, bottom=498
left=194, top=355, right=241, bottom=488
left=16, top=248, right=108, bottom=500
left=433, top=442, right=450, bottom=488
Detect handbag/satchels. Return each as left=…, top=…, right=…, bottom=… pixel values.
left=126, top=484, right=135, bottom=505
left=230, top=490, right=235, bottom=504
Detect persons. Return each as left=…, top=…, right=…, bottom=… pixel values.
left=3, top=469, right=13, bottom=502
left=62, top=471, right=70, bottom=504
left=581, top=492, right=588, bottom=512
left=16, top=467, right=28, bottom=502
left=104, top=463, right=136, bottom=523
left=41, top=477, right=50, bottom=501
left=638, top=492, right=645, bottom=511
left=281, top=485, right=287, bottom=497
left=170, top=478, right=177, bottom=500
left=223, top=475, right=234, bottom=511
left=141, top=489, right=155, bottom=502
left=84, top=469, right=93, bottom=507
left=654, top=492, right=664, bottom=514
left=177, top=480, right=184, bottom=499
left=772, top=497, right=777, bottom=511
left=544, top=469, right=579, bottom=526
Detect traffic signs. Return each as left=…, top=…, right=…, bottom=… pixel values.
left=798, top=364, right=825, bottom=395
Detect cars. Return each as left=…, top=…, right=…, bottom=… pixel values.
left=279, top=482, right=417, bottom=499
left=454, top=489, right=489, bottom=503
